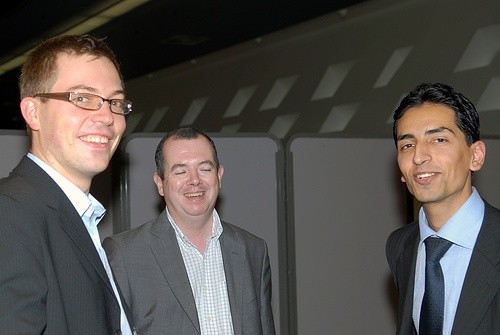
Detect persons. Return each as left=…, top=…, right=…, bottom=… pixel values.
left=386, top=82, right=499, bottom=334
left=104, top=126, right=277, bottom=334
left=1, top=34, right=135, bottom=334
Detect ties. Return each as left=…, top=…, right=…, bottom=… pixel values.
left=418, top=237, right=453, bottom=335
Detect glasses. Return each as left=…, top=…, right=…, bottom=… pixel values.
left=32, top=92, right=132, bottom=115
